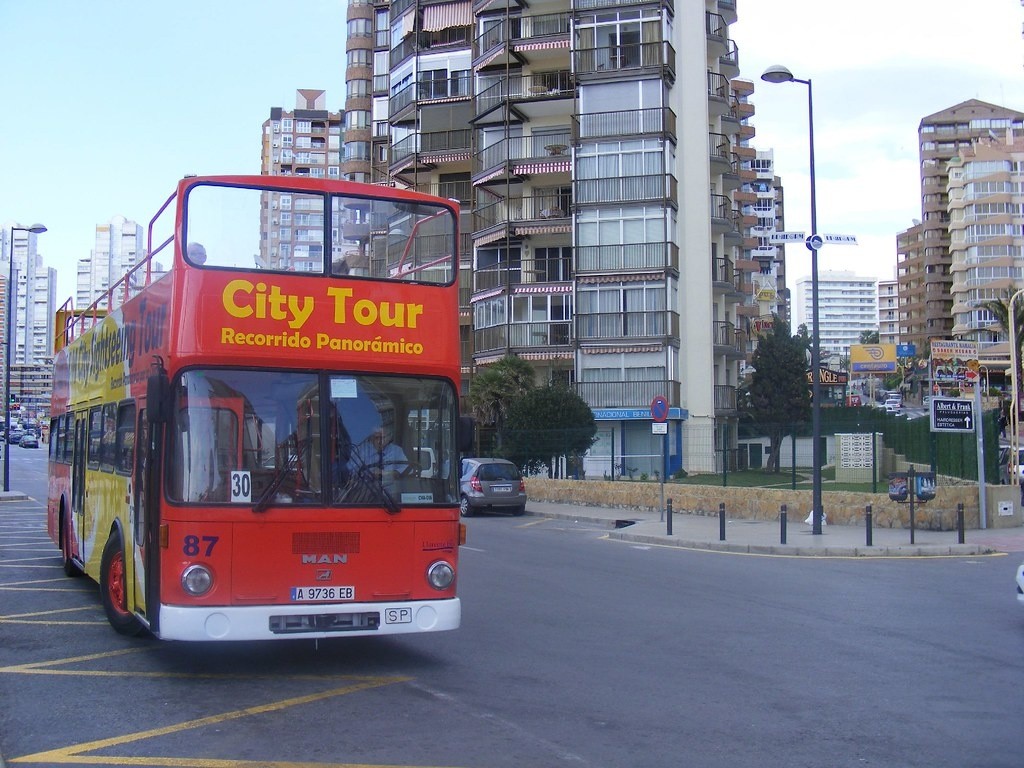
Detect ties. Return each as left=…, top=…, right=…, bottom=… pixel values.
left=379, top=451, right=384, bottom=470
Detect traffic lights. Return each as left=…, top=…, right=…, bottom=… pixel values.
left=11, top=393, right=16, bottom=403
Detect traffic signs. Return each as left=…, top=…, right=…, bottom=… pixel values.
left=928, top=396, right=975, bottom=433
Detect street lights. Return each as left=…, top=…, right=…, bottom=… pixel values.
left=3, top=223, right=47, bottom=492
left=758, top=64, right=823, bottom=535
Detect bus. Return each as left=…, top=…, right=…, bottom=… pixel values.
left=46, top=175, right=467, bottom=642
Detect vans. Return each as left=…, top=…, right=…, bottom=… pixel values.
left=413, top=448, right=437, bottom=478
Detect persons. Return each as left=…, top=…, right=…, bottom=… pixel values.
left=346, top=418, right=413, bottom=479
left=187, top=242, right=207, bottom=266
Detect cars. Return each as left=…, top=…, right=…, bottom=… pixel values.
left=441, top=457, right=527, bottom=518
left=262, top=455, right=302, bottom=472
left=0, top=428, right=38, bottom=449
left=844, top=387, right=904, bottom=417
left=997, top=444, right=1024, bottom=486
